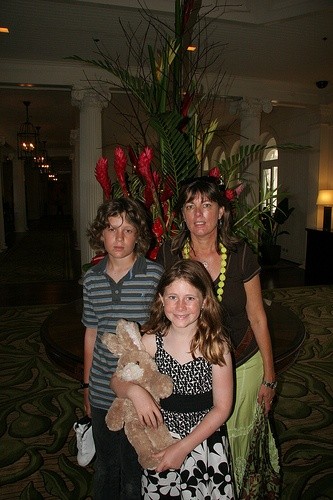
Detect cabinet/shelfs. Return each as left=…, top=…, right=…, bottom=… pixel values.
left=304, top=228, right=333, bottom=286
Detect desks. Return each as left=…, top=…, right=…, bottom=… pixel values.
left=40, top=295, right=306, bottom=384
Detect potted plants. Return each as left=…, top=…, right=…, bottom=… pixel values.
left=260, top=198, right=296, bottom=264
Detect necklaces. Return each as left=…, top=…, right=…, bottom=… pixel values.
left=202, top=259, right=208, bottom=270
left=183, top=240, right=227, bottom=303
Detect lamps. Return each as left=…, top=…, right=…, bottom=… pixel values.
left=15, top=101, right=58, bottom=181
left=317, top=189, right=333, bottom=229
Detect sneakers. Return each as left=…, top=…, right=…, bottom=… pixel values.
left=73, top=416, right=96, bottom=466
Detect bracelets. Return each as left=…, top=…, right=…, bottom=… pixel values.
left=82, top=382, right=88, bottom=388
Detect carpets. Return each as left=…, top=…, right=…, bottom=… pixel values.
left=0, top=221, right=73, bottom=283
left=0, top=287, right=333, bottom=500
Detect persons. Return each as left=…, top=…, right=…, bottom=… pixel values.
left=78, top=197, right=164, bottom=500
left=166, top=175, right=282, bottom=500
left=111, top=261, right=236, bottom=500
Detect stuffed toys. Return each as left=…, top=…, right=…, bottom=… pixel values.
left=102, top=320, right=177, bottom=468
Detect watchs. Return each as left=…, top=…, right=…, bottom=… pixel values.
left=262, top=377, right=279, bottom=390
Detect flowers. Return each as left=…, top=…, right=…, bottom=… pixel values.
left=62, top=0, right=291, bottom=270
